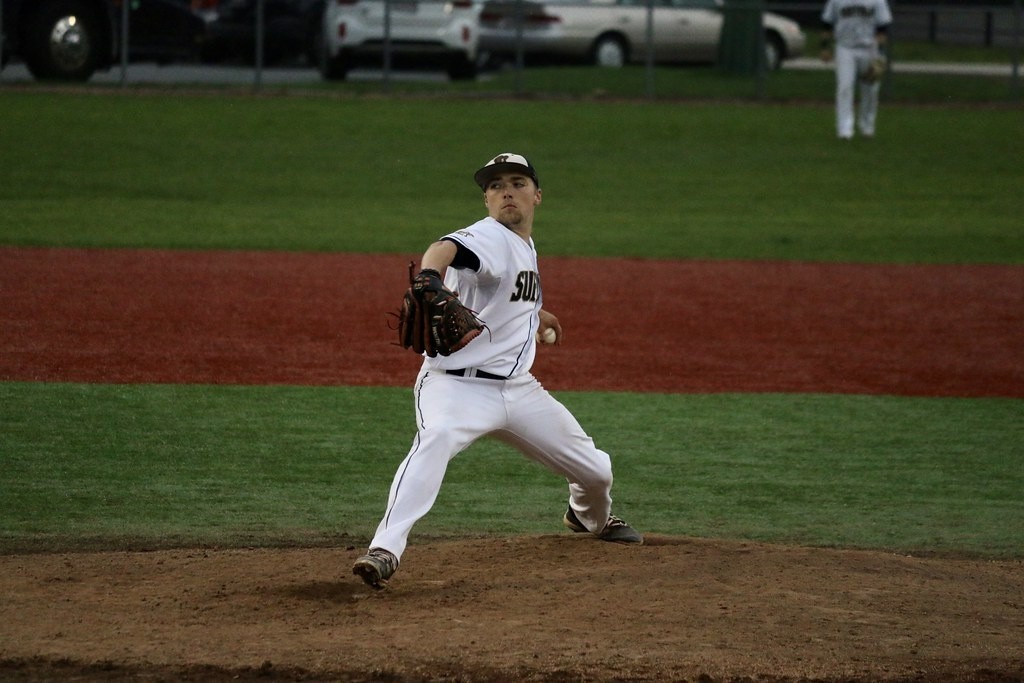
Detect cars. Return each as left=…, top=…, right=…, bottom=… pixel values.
left=0, top=0, right=805, bottom=81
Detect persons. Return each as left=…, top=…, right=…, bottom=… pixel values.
left=823, top=0, right=893, bottom=144
left=351, top=152, right=644, bottom=588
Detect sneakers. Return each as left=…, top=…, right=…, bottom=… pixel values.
left=562, top=505, right=644, bottom=546
left=354, top=548, right=400, bottom=587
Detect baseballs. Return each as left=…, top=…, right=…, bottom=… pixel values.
left=536, top=326, right=557, bottom=345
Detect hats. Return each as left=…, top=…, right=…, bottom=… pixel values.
left=474, top=151, right=540, bottom=192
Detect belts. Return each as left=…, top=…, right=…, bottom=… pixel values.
left=444, top=366, right=506, bottom=381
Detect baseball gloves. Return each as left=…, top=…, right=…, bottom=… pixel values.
left=395, top=267, right=485, bottom=359
